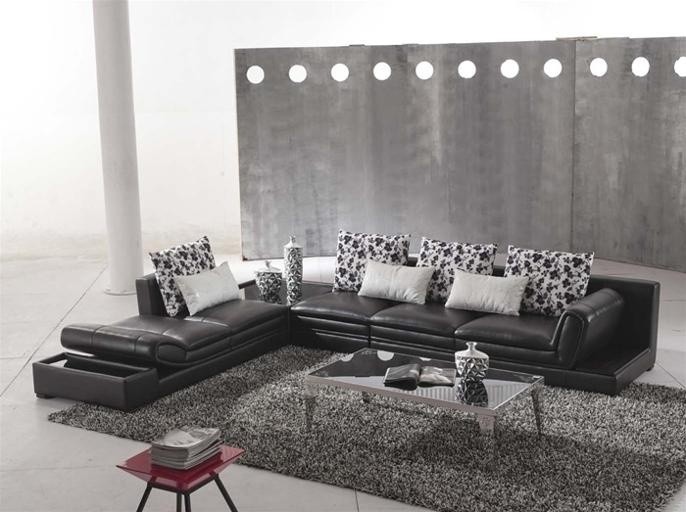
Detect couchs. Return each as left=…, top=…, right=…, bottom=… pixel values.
left=31, top=256, right=660, bottom=411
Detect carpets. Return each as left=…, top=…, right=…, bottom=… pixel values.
left=46, top=343, right=685, bottom=512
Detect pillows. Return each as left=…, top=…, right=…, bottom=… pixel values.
left=145, top=235, right=217, bottom=319
left=332, top=228, right=414, bottom=296
left=442, top=266, right=529, bottom=317
left=357, top=257, right=436, bottom=306
left=408, top=235, right=499, bottom=306
left=171, top=261, right=244, bottom=316
left=503, top=243, right=596, bottom=318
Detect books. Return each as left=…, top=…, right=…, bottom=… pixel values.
left=383, top=363, right=457, bottom=387
left=150, top=423, right=223, bottom=470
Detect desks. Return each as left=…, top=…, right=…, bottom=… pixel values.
left=238, top=279, right=334, bottom=309
left=113, top=443, right=246, bottom=511
